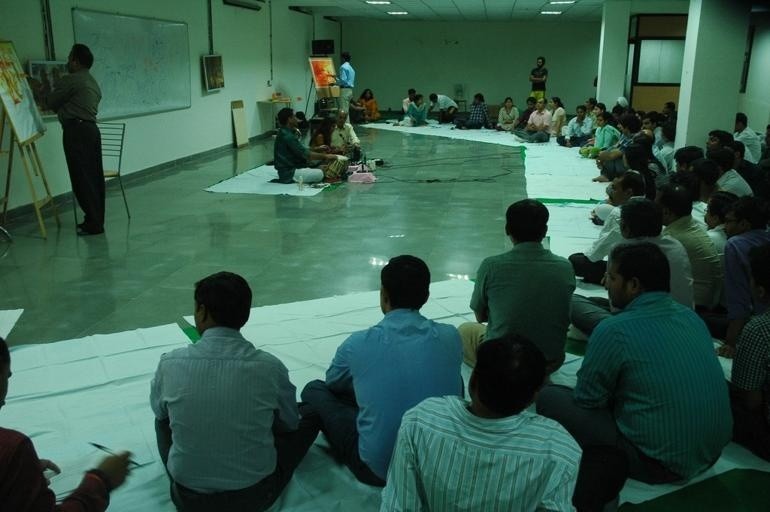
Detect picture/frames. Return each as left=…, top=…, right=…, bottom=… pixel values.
left=202, top=55, right=225, bottom=92
left=27, top=60, right=69, bottom=115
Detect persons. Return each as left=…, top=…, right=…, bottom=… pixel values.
left=568, top=172, right=646, bottom=284
left=557, top=105, right=593, bottom=147
left=732, top=244, right=770, bottom=464
left=309, top=117, right=347, bottom=155
left=429, top=93, right=458, bottom=125
left=536, top=241, right=734, bottom=486
left=0, top=337, right=133, bottom=512
left=515, top=98, right=553, bottom=144
left=378, top=328, right=582, bottom=510
left=570, top=201, right=694, bottom=336
left=149, top=271, right=320, bottom=511
left=495, top=97, right=520, bottom=131
left=634, top=101, right=677, bottom=168
left=510, top=97, right=536, bottom=131
left=579, top=115, right=621, bottom=158
left=453, top=93, right=493, bottom=130
left=327, top=52, right=355, bottom=123
left=728, top=141, right=770, bottom=197
left=300, top=254, right=464, bottom=488
left=592, top=113, right=642, bottom=183
left=583, top=97, right=635, bottom=117
left=402, top=88, right=416, bottom=113
left=716, top=197, right=770, bottom=358
left=733, top=112, right=762, bottom=174
left=274, top=108, right=337, bottom=184
left=549, top=96, right=566, bottom=137
left=622, top=146, right=657, bottom=200
left=29, top=43, right=107, bottom=236
left=529, top=56, right=548, bottom=100
left=706, top=130, right=735, bottom=147
left=358, top=89, right=380, bottom=121
left=703, top=191, right=739, bottom=333
left=457, top=199, right=578, bottom=388
left=331, top=109, right=361, bottom=164
left=393, top=94, right=427, bottom=128
left=706, top=145, right=753, bottom=198
left=652, top=182, right=723, bottom=318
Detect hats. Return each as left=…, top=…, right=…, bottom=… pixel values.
left=617, top=97, right=629, bottom=108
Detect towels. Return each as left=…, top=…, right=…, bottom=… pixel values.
left=336, top=154, right=351, bottom=161
left=348, top=173, right=376, bottom=183
left=358, top=164, right=368, bottom=173
left=346, top=166, right=360, bottom=173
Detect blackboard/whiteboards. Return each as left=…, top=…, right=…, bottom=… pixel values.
left=72, top=7, right=191, bottom=122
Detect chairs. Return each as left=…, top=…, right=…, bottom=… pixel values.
left=69, top=121, right=131, bottom=227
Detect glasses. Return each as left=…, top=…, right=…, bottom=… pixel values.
left=536, top=102, right=543, bottom=104
left=723, top=217, right=738, bottom=225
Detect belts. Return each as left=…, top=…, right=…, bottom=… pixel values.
left=340, top=85, right=353, bottom=89
left=62, top=119, right=97, bottom=126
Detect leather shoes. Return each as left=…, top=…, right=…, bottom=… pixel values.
left=77, top=224, right=105, bottom=235
left=76, top=221, right=87, bottom=228
